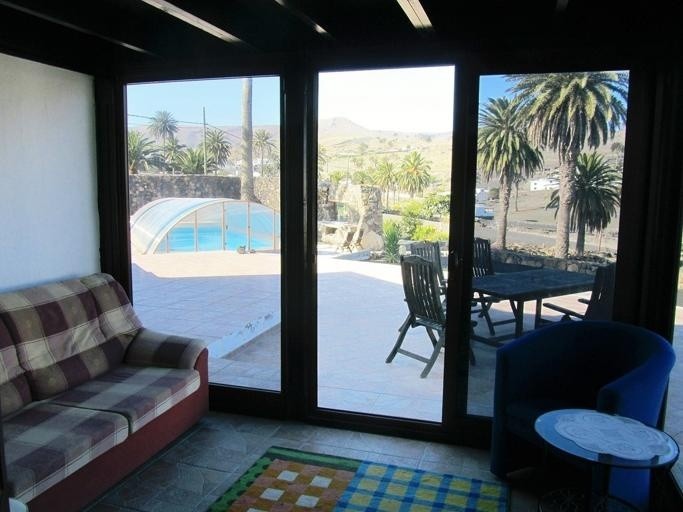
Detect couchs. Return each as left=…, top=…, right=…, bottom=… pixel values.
left=0, top=272, right=210, bottom=512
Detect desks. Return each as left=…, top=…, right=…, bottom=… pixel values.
left=533, top=408, right=680, bottom=512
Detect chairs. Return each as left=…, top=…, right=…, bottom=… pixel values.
left=385, top=236, right=616, bottom=379
left=335, top=227, right=366, bottom=253
left=490, top=318, right=677, bottom=512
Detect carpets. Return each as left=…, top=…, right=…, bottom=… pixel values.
left=204, top=445, right=511, bottom=512
